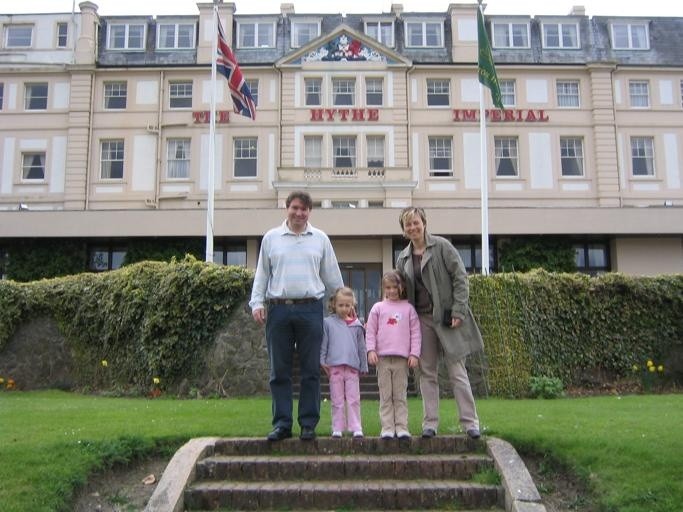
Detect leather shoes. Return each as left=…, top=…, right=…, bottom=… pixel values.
left=268, top=427, right=292, bottom=440
left=300, top=426, right=315, bottom=440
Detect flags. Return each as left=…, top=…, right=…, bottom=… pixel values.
left=216, top=12, right=256, bottom=121
left=477, top=6, right=506, bottom=114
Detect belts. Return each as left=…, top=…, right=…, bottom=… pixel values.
left=270, top=298, right=314, bottom=304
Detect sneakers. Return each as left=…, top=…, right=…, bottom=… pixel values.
left=422, top=429, right=435, bottom=438
left=332, top=431, right=342, bottom=437
left=353, top=431, right=363, bottom=438
left=397, top=431, right=411, bottom=438
left=467, top=430, right=480, bottom=437
left=381, top=430, right=394, bottom=438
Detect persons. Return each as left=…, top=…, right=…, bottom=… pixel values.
left=320, top=287, right=369, bottom=437
left=393, top=205, right=486, bottom=438
left=248, top=190, right=344, bottom=441
left=364, top=271, right=423, bottom=438
